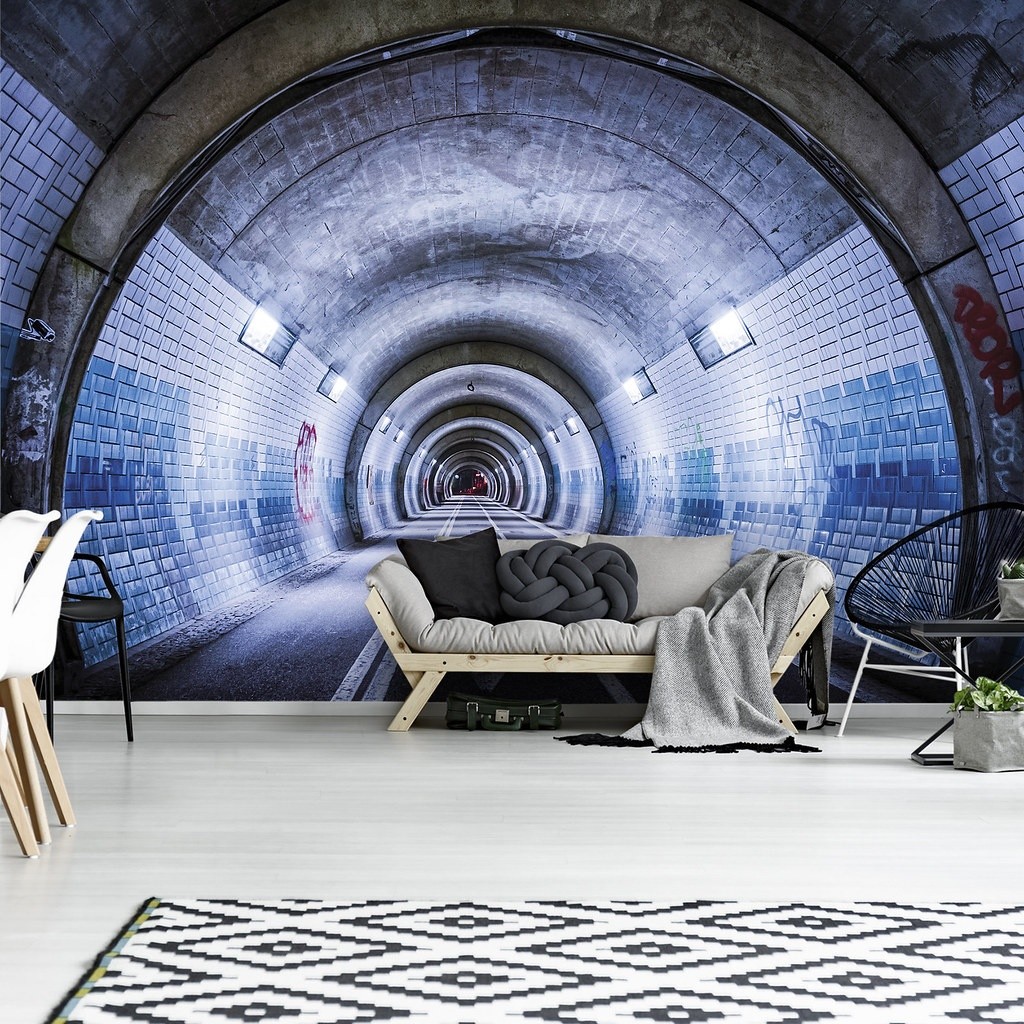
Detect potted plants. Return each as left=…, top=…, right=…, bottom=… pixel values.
left=993, top=564, right=1024, bottom=621
left=947, top=676, right=1023, bottom=772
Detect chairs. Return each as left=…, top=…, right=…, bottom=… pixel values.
left=834, top=502, right=1024, bottom=738
left=0, top=508, right=105, bottom=864
left=28, top=538, right=133, bottom=745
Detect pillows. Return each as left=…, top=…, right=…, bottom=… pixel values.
left=434, top=534, right=590, bottom=557
left=587, top=535, right=735, bottom=621
left=396, top=526, right=511, bottom=625
left=365, top=535, right=834, bottom=732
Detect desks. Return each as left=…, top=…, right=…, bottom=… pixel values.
left=909, top=621, right=1024, bottom=765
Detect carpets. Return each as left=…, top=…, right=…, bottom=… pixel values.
left=41, top=894, right=1023, bottom=1024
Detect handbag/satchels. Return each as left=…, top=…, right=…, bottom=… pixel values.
left=444, top=692, right=563, bottom=731
